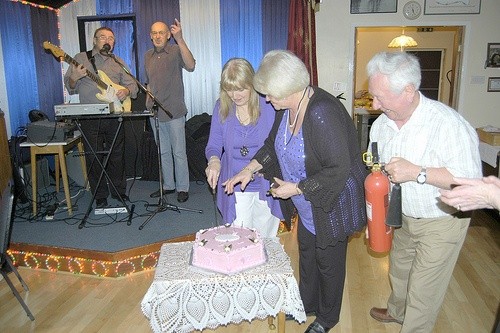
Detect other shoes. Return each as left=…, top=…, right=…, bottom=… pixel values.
left=95, top=198, right=107, bottom=207
left=111, top=193, right=129, bottom=201
left=177, top=192, right=189, bottom=203
left=285, top=310, right=307, bottom=320
left=150, top=188, right=175, bottom=198
left=304, top=318, right=329, bottom=333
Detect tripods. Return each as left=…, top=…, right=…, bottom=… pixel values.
left=105, top=50, right=203, bottom=230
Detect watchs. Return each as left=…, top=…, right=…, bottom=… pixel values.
left=416, top=166, right=427, bottom=184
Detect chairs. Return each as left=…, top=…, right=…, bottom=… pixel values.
left=0, top=183, right=35, bottom=319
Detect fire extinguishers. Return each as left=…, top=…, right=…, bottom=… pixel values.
left=363, top=142, right=402, bottom=258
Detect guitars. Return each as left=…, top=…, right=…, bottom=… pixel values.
left=43, top=39, right=131, bottom=114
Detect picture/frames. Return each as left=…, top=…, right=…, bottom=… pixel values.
left=349, top=0, right=398, bottom=15
left=487, top=42, right=500, bottom=68
left=487, top=76, right=500, bottom=92
left=424, top=0, right=481, bottom=15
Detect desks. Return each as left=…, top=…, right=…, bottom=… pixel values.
left=52, top=112, right=155, bottom=229
left=19, top=133, right=90, bottom=218
left=140, top=237, right=308, bottom=333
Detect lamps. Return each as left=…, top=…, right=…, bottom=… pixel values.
left=388, top=29, right=417, bottom=53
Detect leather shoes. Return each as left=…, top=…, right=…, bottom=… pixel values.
left=370, top=307, right=396, bottom=322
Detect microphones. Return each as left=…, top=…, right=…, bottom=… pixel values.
left=99, top=43, right=111, bottom=52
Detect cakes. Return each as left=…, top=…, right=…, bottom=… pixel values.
left=192, top=223, right=266, bottom=273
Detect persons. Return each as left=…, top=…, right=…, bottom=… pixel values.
left=367, top=52, right=483, bottom=333
left=205, top=57, right=285, bottom=237
left=221, top=49, right=372, bottom=333
left=143, top=18, right=196, bottom=203
left=438, top=175, right=500, bottom=215
left=64, top=27, right=140, bottom=209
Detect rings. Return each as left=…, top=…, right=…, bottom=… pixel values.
left=390, top=175, right=393, bottom=179
left=457, top=205, right=461, bottom=211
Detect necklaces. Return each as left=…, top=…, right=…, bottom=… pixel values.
left=240, top=144, right=250, bottom=157
left=284, top=85, right=308, bottom=146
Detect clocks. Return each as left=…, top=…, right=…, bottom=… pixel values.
left=403, top=1, right=422, bottom=20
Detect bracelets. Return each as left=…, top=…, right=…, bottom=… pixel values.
left=240, top=167, right=254, bottom=181
left=295, top=182, right=301, bottom=194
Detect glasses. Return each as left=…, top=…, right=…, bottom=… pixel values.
left=150, top=31, right=168, bottom=35
left=96, top=36, right=114, bottom=42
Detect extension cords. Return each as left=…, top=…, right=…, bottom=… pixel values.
left=46, top=208, right=59, bottom=220
left=95, top=206, right=127, bottom=215
